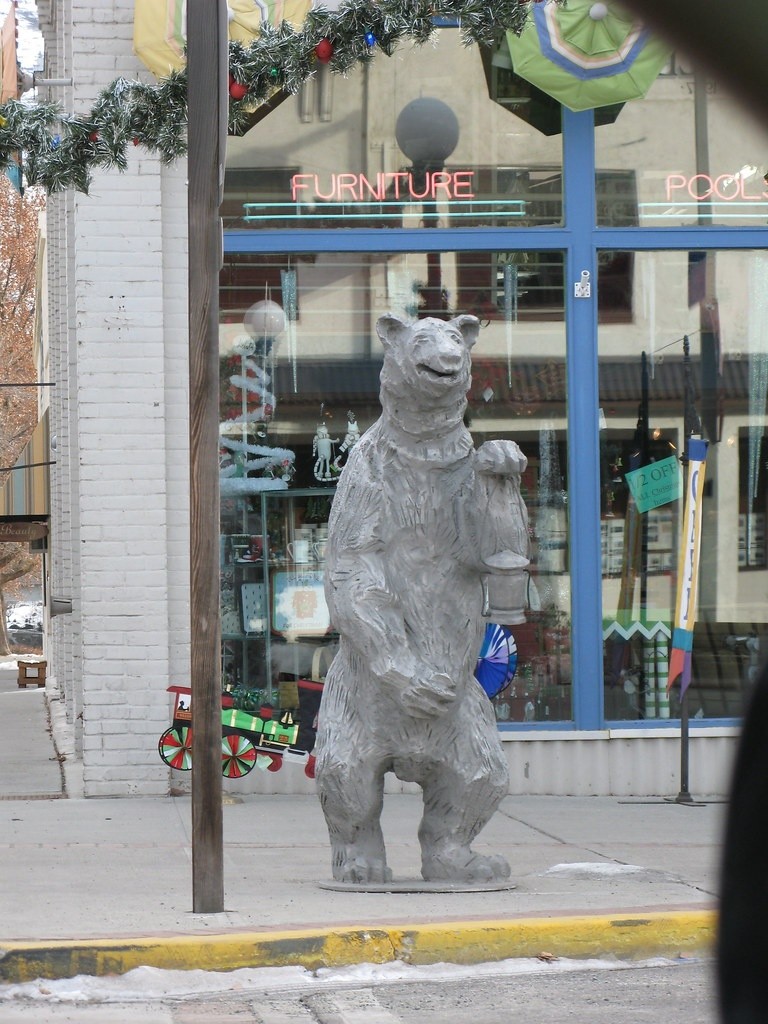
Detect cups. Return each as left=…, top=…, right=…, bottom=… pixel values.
left=313, top=539, right=328, bottom=562
left=288, top=540, right=309, bottom=563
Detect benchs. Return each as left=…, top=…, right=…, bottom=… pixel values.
left=17, top=660, right=47, bottom=688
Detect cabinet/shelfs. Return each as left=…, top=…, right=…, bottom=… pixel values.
left=216, top=485, right=337, bottom=712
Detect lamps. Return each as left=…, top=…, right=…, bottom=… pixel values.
left=479, top=477, right=531, bottom=625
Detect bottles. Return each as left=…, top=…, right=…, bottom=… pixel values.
left=534, top=674, right=549, bottom=720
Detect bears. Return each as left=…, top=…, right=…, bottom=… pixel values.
left=314, top=316, right=531, bottom=885
